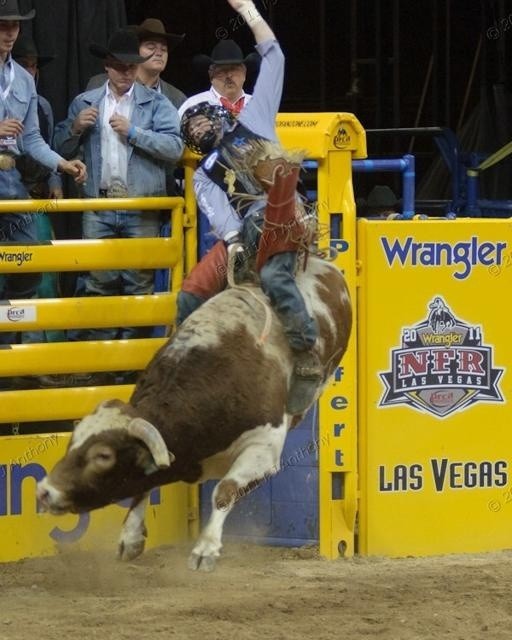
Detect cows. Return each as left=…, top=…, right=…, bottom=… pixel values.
left=35, top=255, right=353, bottom=572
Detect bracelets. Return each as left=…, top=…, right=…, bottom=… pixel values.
left=234, top=2, right=263, bottom=28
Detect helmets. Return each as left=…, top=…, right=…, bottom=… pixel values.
left=181, top=102, right=234, bottom=154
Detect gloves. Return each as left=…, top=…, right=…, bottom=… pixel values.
left=224, top=230, right=248, bottom=283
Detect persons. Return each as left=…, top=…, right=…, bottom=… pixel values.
left=177, top=40, right=254, bottom=124
left=0, top=0, right=88, bottom=386
left=358, top=184, right=403, bottom=218
left=85, top=17, right=187, bottom=114
left=11, top=32, right=66, bottom=198
left=51, top=28, right=185, bottom=385
left=172, top=0, right=330, bottom=416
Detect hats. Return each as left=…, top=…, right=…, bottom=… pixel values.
left=10, top=36, right=39, bottom=57
left=89, top=30, right=156, bottom=62
left=0, top=0, right=35, bottom=20
left=125, top=19, right=185, bottom=43
left=193, top=40, right=262, bottom=64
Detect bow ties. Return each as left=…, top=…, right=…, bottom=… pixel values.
left=222, top=96, right=245, bottom=112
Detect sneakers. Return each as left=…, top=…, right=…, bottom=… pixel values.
left=285, top=352, right=323, bottom=416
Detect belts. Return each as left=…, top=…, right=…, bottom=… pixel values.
left=97, top=189, right=126, bottom=197
